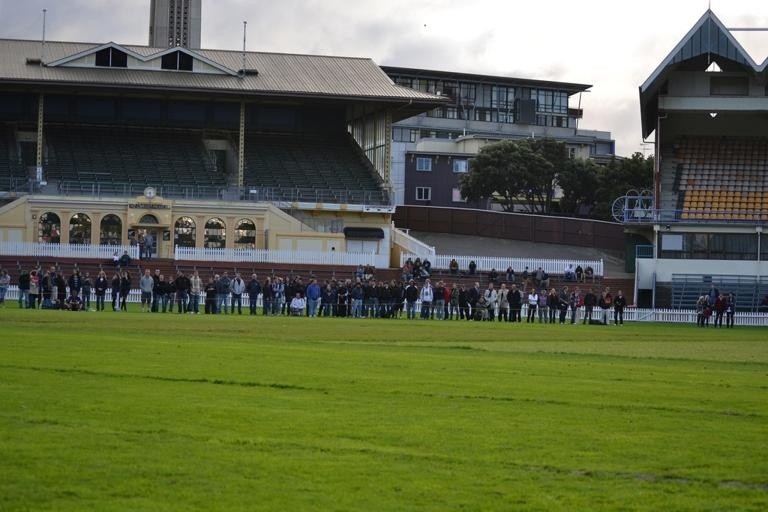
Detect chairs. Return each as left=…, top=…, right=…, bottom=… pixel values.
left=672, top=138, right=768, bottom=222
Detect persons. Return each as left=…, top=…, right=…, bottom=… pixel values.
left=759, top=295, right=768, bottom=312
left=0, top=268, right=11, bottom=303
left=695, top=282, right=736, bottom=328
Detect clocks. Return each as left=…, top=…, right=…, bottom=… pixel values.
left=144, top=186, right=157, bottom=197
